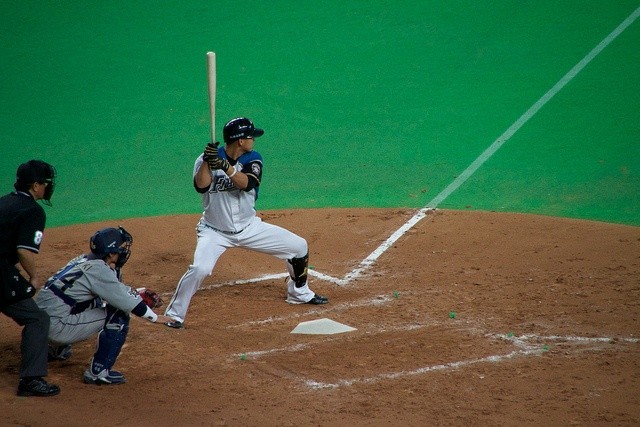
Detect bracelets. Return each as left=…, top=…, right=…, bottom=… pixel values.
left=229, top=165, right=237, bottom=178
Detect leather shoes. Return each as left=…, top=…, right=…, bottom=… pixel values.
left=306, top=293, right=329, bottom=305
left=165, top=320, right=184, bottom=329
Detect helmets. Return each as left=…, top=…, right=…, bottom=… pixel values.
left=223, top=117, right=264, bottom=143
left=14, top=159, right=58, bottom=207
left=86, top=227, right=134, bottom=269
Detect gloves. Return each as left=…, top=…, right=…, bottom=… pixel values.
left=208, top=156, right=230, bottom=173
left=203, top=141, right=220, bottom=162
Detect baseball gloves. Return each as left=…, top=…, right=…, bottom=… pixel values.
left=135, top=287, right=164, bottom=309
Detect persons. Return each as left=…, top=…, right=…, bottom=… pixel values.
left=34, top=224, right=176, bottom=386
left=0, top=159, right=60, bottom=397
left=163, top=116, right=329, bottom=329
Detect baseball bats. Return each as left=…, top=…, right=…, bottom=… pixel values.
left=207, top=52, right=216, bottom=144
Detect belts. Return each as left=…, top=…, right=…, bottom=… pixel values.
left=204, top=222, right=252, bottom=235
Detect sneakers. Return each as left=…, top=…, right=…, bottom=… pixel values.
left=17, top=378, right=61, bottom=398
left=48, top=343, right=72, bottom=361
left=83, top=361, right=126, bottom=385
